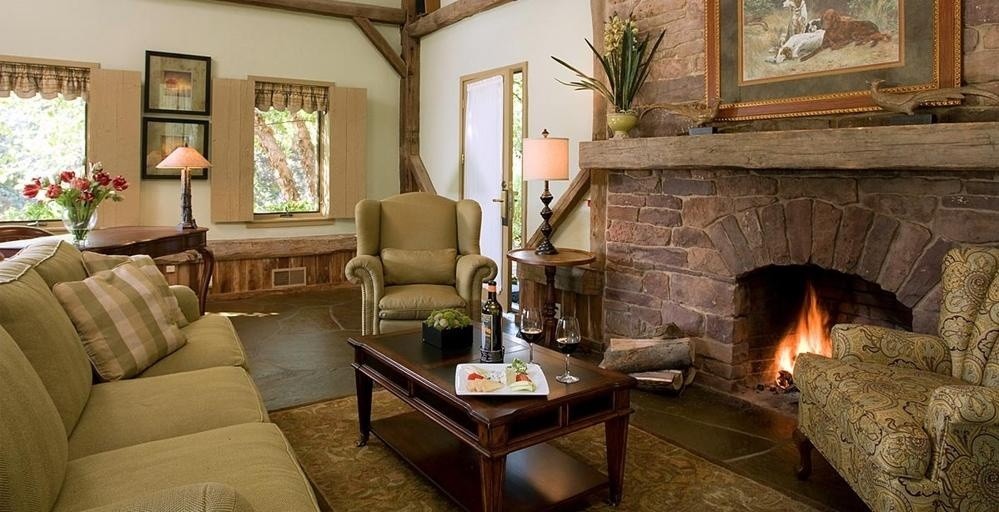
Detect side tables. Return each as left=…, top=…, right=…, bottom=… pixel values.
left=507, top=248, right=596, bottom=347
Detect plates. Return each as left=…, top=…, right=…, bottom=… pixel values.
left=454, top=362, right=551, bottom=397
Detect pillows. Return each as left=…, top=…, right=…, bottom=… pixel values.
left=81, top=251, right=188, bottom=328
left=381, top=248, right=457, bottom=285
left=52, top=257, right=188, bottom=382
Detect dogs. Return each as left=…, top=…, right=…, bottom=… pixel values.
left=801, top=16, right=856, bottom=34
left=775, top=29, right=826, bottom=64
left=800, top=8, right=891, bottom=62
left=775, top=0, right=808, bottom=50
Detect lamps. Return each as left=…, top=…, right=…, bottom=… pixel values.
left=523, top=129, right=569, bottom=255
left=155, top=143, right=214, bottom=229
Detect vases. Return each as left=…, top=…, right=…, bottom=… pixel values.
left=422, top=322, right=473, bottom=350
left=607, top=112, right=637, bottom=139
left=61, top=207, right=97, bottom=246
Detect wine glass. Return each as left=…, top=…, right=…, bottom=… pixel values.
left=519, top=303, right=544, bottom=369
left=554, top=316, right=582, bottom=385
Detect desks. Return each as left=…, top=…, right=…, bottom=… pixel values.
left=0, top=224, right=215, bottom=316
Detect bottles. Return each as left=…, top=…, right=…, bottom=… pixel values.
left=480, top=281, right=503, bottom=363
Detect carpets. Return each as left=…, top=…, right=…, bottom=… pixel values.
left=268, top=386, right=817, bottom=512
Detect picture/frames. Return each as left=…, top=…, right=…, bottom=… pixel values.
left=144, top=50, right=211, bottom=115
left=704, top=0, right=962, bottom=120
left=142, top=116, right=209, bottom=179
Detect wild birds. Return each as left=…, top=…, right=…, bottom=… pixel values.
left=638, top=96, right=725, bottom=128
left=865, top=79, right=999, bottom=116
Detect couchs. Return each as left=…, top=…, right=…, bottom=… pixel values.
left=0, top=240, right=320, bottom=512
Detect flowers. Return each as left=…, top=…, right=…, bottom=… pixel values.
left=23, top=160, right=127, bottom=239
left=425, top=308, right=470, bottom=332
left=551, top=10, right=667, bottom=112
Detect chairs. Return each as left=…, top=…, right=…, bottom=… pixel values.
left=0, top=226, right=54, bottom=261
left=345, top=192, right=498, bottom=335
left=791, top=246, right=999, bottom=512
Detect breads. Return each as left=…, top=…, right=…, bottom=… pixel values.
left=467, top=380, right=503, bottom=392
left=508, top=381, right=535, bottom=392
